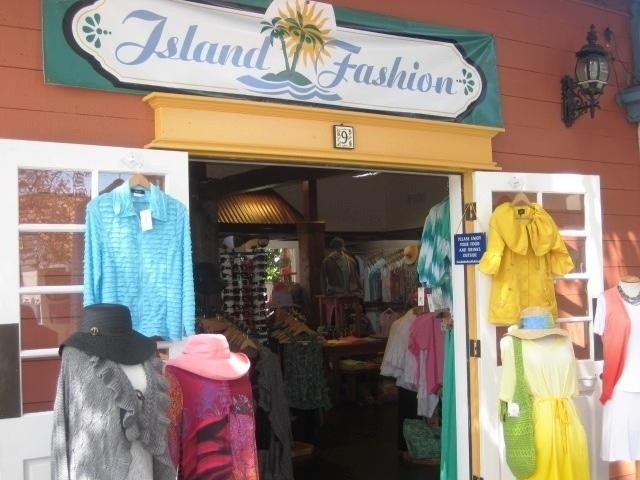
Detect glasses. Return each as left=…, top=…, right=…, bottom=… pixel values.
left=216, top=255, right=270, bottom=347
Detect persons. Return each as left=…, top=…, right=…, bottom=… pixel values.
left=159, top=333, right=259, bottom=479
left=496, top=306, right=592, bottom=480
left=319, top=235, right=364, bottom=299
left=50, top=302, right=181, bottom=479
left=593, top=275, right=639, bottom=480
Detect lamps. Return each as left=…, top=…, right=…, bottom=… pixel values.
left=560, top=24, right=610, bottom=128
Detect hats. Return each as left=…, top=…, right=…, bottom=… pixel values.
left=329, top=237, right=344, bottom=248
left=507, top=307, right=569, bottom=340
left=274, top=258, right=291, bottom=276
left=404, top=245, right=418, bottom=264
left=162, top=333, right=251, bottom=380
left=268, top=283, right=302, bottom=308
left=194, top=262, right=224, bottom=293
left=59, top=304, right=157, bottom=365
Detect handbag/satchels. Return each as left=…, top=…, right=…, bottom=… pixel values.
left=502, top=379, right=536, bottom=479
left=403, top=413, right=441, bottom=459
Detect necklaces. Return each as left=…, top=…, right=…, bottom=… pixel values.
left=617, top=286, right=640, bottom=306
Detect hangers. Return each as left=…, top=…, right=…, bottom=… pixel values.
left=511, top=179, right=534, bottom=209
left=129, top=156, right=149, bottom=189
left=200, top=305, right=328, bottom=353
left=365, top=248, right=404, bottom=270
